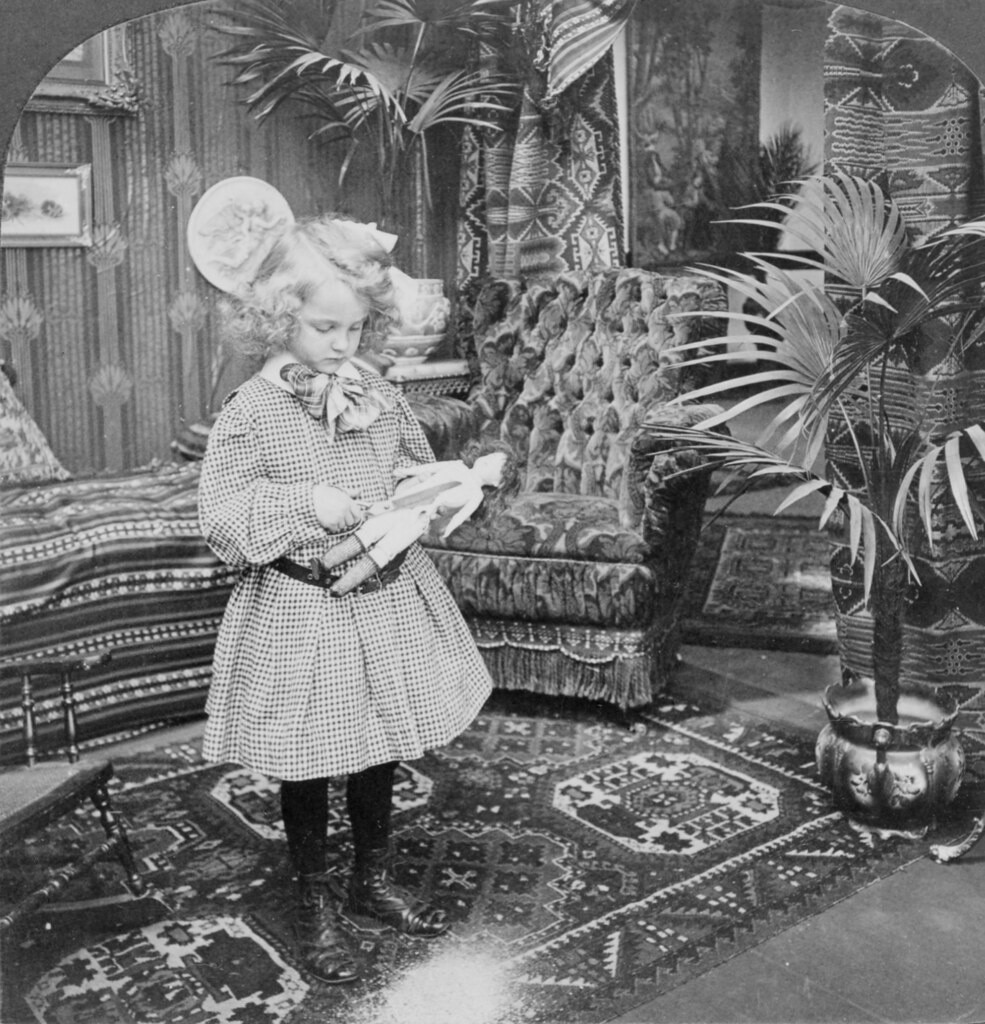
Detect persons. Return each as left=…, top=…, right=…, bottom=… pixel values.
left=199, top=212, right=495, bottom=984
left=310, top=435, right=519, bottom=598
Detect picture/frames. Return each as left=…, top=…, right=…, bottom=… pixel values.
left=23, top=20, right=138, bottom=114
left=1, top=162, right=93, bottom=248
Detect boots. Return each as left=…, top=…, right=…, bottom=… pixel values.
left=282, top=867, right=360, bottom=984
left=349, top=847, right=450, bottom=938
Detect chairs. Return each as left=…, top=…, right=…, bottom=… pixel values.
left=0, top=647, right=147, bottom=932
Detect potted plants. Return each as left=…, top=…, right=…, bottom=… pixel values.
left=631, top=165, right=985, bottom=830
left=198, top=0, right=515, bottom=367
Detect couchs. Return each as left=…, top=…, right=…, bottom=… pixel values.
left=404, top=267, right=729, bottom=711
left=0, top=363, right=252, bottom=774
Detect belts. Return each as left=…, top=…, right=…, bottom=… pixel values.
left=268, top=544, right=411, bottom=597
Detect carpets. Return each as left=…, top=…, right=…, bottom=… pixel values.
left=0, top=660, right=985, bottom=1024
left=681, top=510, right=846, bottom=655
